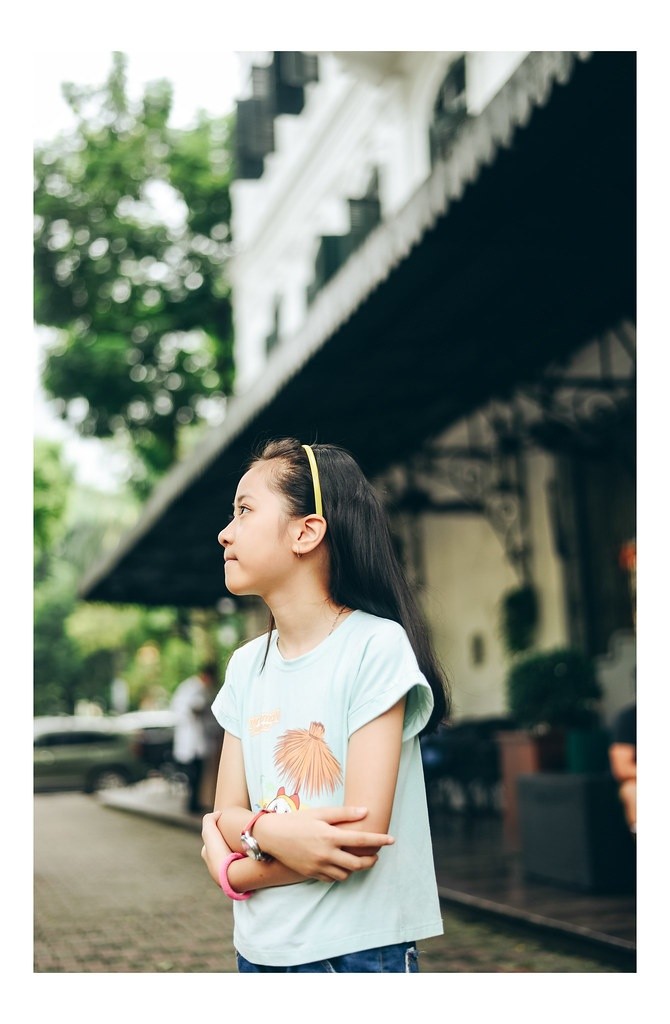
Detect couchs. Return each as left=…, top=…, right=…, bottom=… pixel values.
left=516, top=728, right=637, bottom=899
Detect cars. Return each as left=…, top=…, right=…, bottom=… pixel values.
left=33, top=716, right=148, bottom=795
left=117, top=709, right=175, bottom=771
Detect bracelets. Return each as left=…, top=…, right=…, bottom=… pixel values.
left=219, top=852, right=253, bottom=900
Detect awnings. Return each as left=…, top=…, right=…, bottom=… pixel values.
left=79, top=51, right=636, bottom=610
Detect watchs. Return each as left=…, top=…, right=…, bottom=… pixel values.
left=241, top=808, right=276, bottom=863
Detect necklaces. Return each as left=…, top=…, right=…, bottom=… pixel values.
left=328, top=605, right=347, bottom=636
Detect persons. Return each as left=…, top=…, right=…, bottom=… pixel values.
left=171, top=662, right=217, bottom=815
left=202, top=439, right=452, bottom=973
